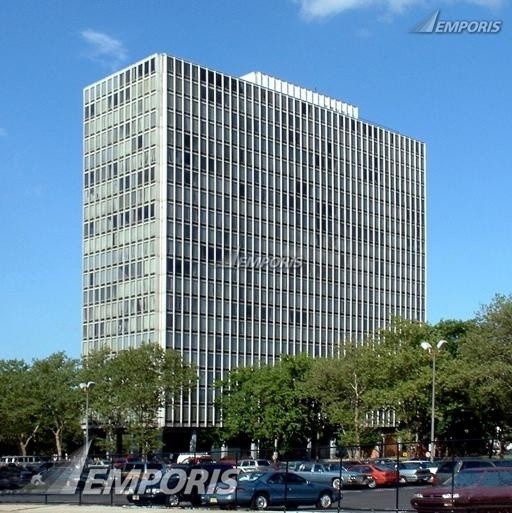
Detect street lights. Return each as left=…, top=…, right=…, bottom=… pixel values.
left=420, top=338, right=450, bottom=459
left=78, top=379, right=96, bottom=454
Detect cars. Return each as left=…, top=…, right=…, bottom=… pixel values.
left=408, top=465, right=512, bottom=513
left=203, top=467, right=342, bottom=508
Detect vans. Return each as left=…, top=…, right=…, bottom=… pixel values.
left=124, top=460, right=235, bottom=505
